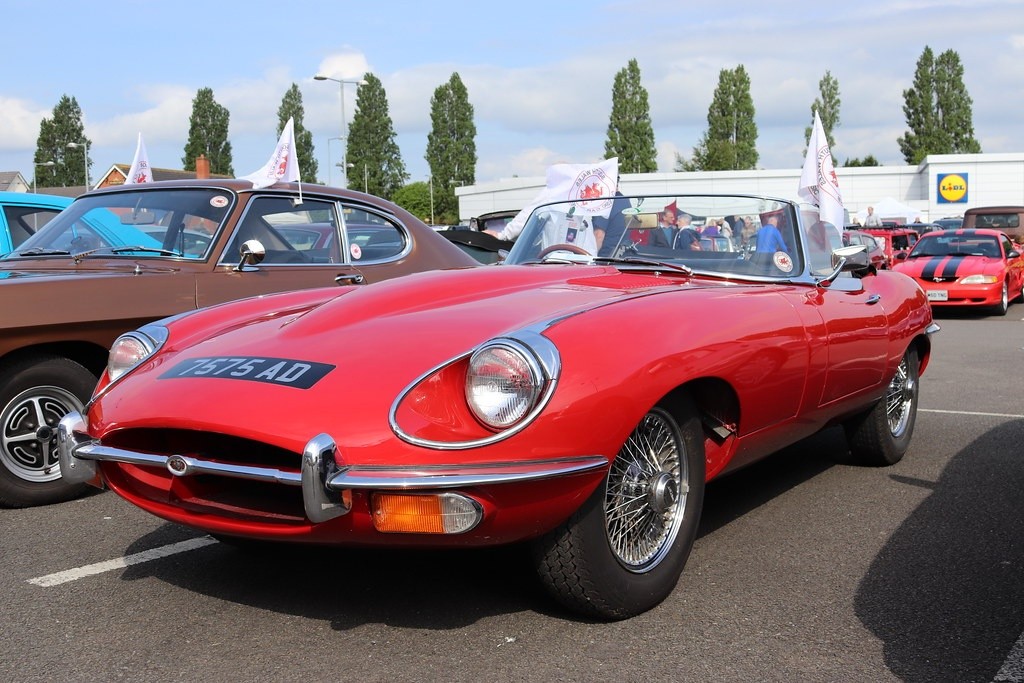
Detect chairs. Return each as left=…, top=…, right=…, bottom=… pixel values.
left=935, top=243, right=950, bottom=255
left=979, top=243, right=997, bottom=259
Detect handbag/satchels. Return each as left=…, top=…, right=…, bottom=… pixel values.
left=679, top=229, right=704, bottom=251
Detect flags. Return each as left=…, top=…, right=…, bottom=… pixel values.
left=798, top=109, right=844, bottom=242
left=123, top=138, right=153, bottom=184
left=237, top=116, right=301, bottom=189
left=535, top=156, right=619, bottom=219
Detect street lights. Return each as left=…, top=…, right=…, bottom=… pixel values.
left=67, top=142, right=89, bottom=193
left=32, top=161, right=56, bottom=234
left=328, top=135, right=348, bottom=186
left=313, top=73, right=369, bottom=220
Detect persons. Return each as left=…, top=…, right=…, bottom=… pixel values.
left=592, top=176, right=632, bottom=260
left=914, top=216, right=922, bottom=224
left=482, top=204, right=598, bottom=263
left=854, top=218, right=861, bottom=228
left=756, top=216, right=790, bottom=253
left=863, top=207, right=883, bottom=227
left=647, top=208, right=755, bottom=254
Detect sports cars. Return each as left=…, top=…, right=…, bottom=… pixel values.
left=890, top=229, right=1024, bottom=316
left=57, top=191, right=942, bottom=624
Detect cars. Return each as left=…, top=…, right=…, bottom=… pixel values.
left=271, top=222, right=401, bottom=251
left=698, top=234, right=757, bottom=254
left=842, top=206, right=1024, bottom=271
left=0, top=190, right=201, bottom=279
left=131, top=224, right=212, bottom=256
left=426, top=210, right=522, bottom=266
left=0, top=177, right=484, bottom=509
